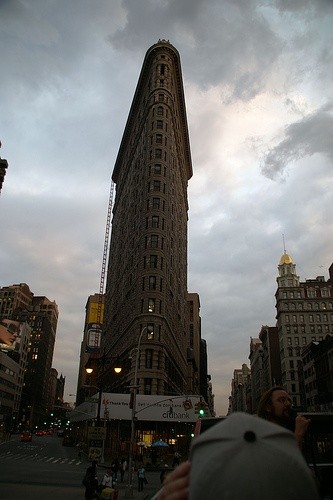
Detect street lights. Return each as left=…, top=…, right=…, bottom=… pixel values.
left=81, top=385, right=107, bottom=448
left=125, top=326, right=153, bottom=499
left=261, top=325, right=273, bottom=389
left=85, top=346, right=122, bottom=446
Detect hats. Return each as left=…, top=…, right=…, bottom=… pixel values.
left=187, top=411, right=317, bottom=500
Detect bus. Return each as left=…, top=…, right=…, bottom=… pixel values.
left=194, top=412, right=333, bottom=498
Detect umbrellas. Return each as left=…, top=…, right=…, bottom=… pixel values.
left=153, top=441, right=169, bottom=448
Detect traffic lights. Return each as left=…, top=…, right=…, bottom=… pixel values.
left=50, top=410, right=54, bottom=418
left=195, top=401, right=209, bottom=415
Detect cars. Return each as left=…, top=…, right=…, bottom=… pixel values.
left=21, top=431, right=33, bottom=441
left=35, top=429, right=52, bottom=436
left=63, top=435, right=74, bottom=446
left=57, top=429, right=64, bottom=437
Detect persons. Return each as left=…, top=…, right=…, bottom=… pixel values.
left=85, top=460, right=98, bottom=500
left=153, top=412, right=318, bottom=500
left=172, top=450, right=182, bottom=467
left=257, top=387, right=310, bottom=448
left=137, top=464, right=145, bottom=492
left=111, top=458, right=119, bottom=480
left=119, top=456, right=127, bottom=482
left=151, top=449, right=157, bottom=464
